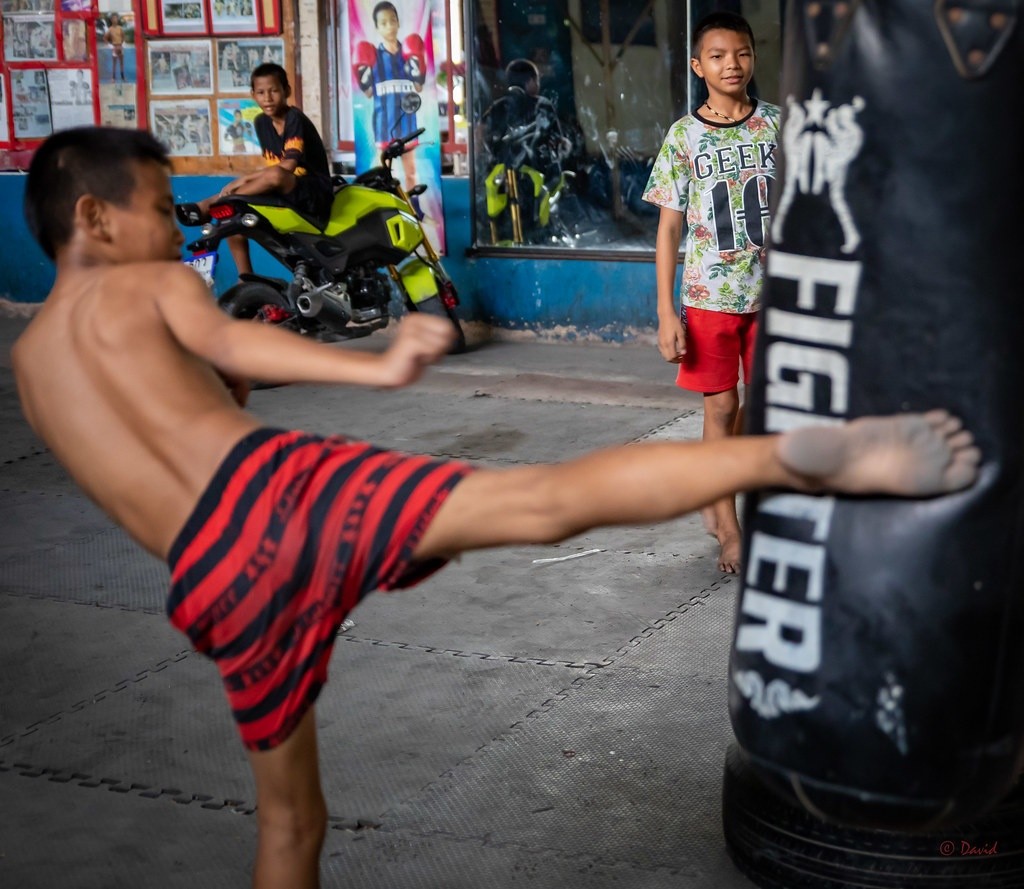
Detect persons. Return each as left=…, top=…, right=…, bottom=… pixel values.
left=174, top=62, right=332, bottom=280
left=0, top=0, right=94, bottom=129
left=101, top=12, right=126, bottom=84
left=150, top=0, right=279, bottom=153
left=10, top=123, right=981, bottom=889
left=503, top=58, right=566, bottom=170
left=354, top=2, right=426, bottom=194
left=640, top=11, right=783, bottom=574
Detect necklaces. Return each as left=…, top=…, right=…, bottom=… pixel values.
left=702, top=99, right=737, bottom=124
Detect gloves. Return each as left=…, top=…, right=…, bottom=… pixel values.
left=351, top=40, right=377, bottom=91
left=401, top=33, right=426, bottom=85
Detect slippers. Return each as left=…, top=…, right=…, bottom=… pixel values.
left=176, top=202, right=212, bottom=227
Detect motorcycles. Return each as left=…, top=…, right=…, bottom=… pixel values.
left=186, top=92, right=466, bottom=390
left=476, top=57, right=576, bottom=240
